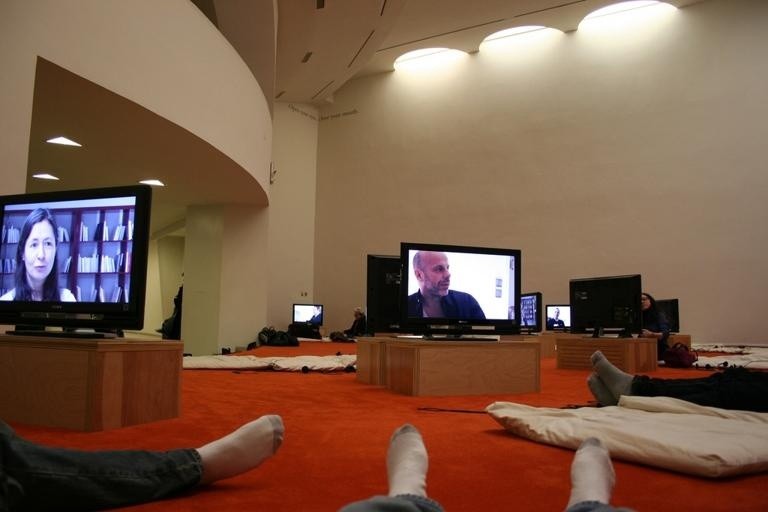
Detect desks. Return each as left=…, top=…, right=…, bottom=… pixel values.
left=1, top=334, right=186, bottom=432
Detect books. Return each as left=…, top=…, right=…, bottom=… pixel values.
left=0, top=207, right=135, bottom=304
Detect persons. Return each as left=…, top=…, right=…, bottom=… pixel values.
left=331, top=423, right=635, bottom=511
left=408, top=251, right=485, bottom=321
left=622, top=292, right=670, bottom=363
left=342, top=306, right=368, bottom=340
left=583, top=349, right=767, bottom=414
left=308, top=305, right=322, bottom=323
left=0, top=412, right=284, bottom=510
left=547, top=307, right=566, bottom=329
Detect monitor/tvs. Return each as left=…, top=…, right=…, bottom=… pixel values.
left=545, top=303, right=572, bottom=333
left=518, top=290, right=542, bottom=336
left=567, top=274, right=644, bottom=338
left=0, top=183, right=151, bottom=340
left=365, top=252, right=404, bottom=335
left=400, top=243, right=521, bottom=339
left=292, top=302, right=324, bottom=328
left=651, top=297, right=680, bottom=333
